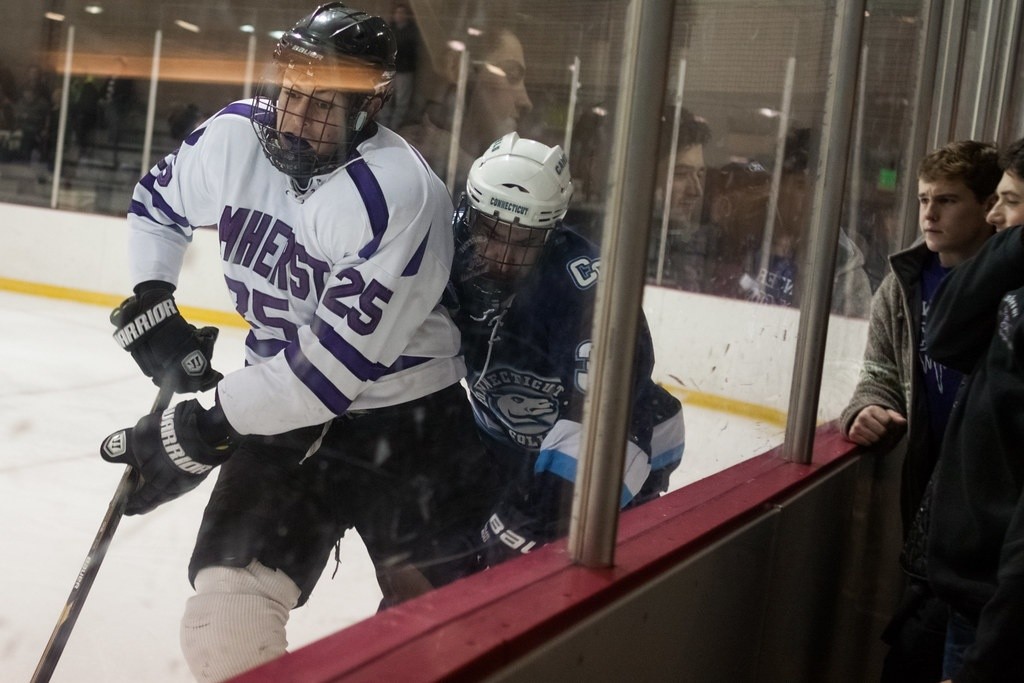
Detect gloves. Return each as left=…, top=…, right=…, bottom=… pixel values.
left=478, top=483, right=571, bottom=567
left=109, top=287, right=223, bottom=393
left=100, top=398, right=239, bottom=517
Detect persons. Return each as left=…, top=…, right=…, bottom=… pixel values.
left=0, top=56, right=134, bottom=174
left=443, top=130, right=685, bottom=573
left=383, top=3, right=418, bottom=129
left=109, top=2, right=489, bottom=683
left=837, top=140, right=1024, bottom=683
left=400, top=18, right=872, bottom=319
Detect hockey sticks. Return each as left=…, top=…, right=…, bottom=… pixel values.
left=28, top=365, right=179, bottom=683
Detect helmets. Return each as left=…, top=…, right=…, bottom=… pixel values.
left=453, top=131, right=573, bottom=298
left=248, top=2, right=400, bottom=179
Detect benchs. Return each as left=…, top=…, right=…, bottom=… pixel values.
left=1, top=113, right=184, bottom=217
